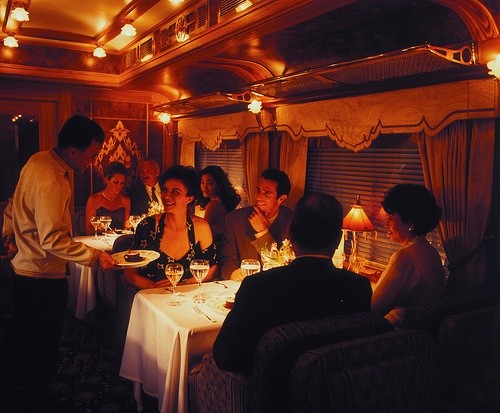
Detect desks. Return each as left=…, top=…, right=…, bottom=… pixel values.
left=61, top=232, right=132, bottom=322
left=119, top=280, right=248, bottom=413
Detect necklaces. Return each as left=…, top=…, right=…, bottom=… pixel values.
left=102, top=191, right=119, bottom=201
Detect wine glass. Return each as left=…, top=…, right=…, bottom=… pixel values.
left=90, top=216, right=101, bottom=240
left=190, top=260, right=210, bottom=304
left=165, top=263, right=184, bottom=305
left=100, top=216, right=112, bottom=242
left=129, top=216, right=141, bottom=234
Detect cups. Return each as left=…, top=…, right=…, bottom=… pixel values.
left=240, top=259, right=261, bottom=278
left=114, top=230, right=122, bottom=234
left=225, top=297, right=236, bottom=309
left=141, top=214, right=151, bottom=220
left=124, top=252, right=141, bottom=262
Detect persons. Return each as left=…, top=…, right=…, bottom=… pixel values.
left=0, top=115, right=124, bottom=413
left=85, top=162, right=295, bottom=323
left=371, top=182, right=447, bottom=329
left=212, top=193, right=374, bottom=372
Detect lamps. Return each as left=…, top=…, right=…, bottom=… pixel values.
left=10, top=2, right=30, bottom=21
left=93, top=41, right=108, bottom=58
left=247, top=99, right=266, bottom=136
left=120, top=19, right=136, bottom=37
left=341, top=193, right=375, bottom=273
left=4, top=30, right=20, bottom=47
left=159, top=113, right=174, bottom=137
left=486, top=54, right=500, bottom=86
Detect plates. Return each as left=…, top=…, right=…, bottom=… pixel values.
left=102, top=229, right=134, bottom=236
left=111, top=250, right=161, bottom=268
left=205, top=294, right=235, bottom=315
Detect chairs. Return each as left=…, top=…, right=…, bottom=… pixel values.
left=112, top=233, right=138, bottom=338
left=185, top=284, right=500, bottom=413
